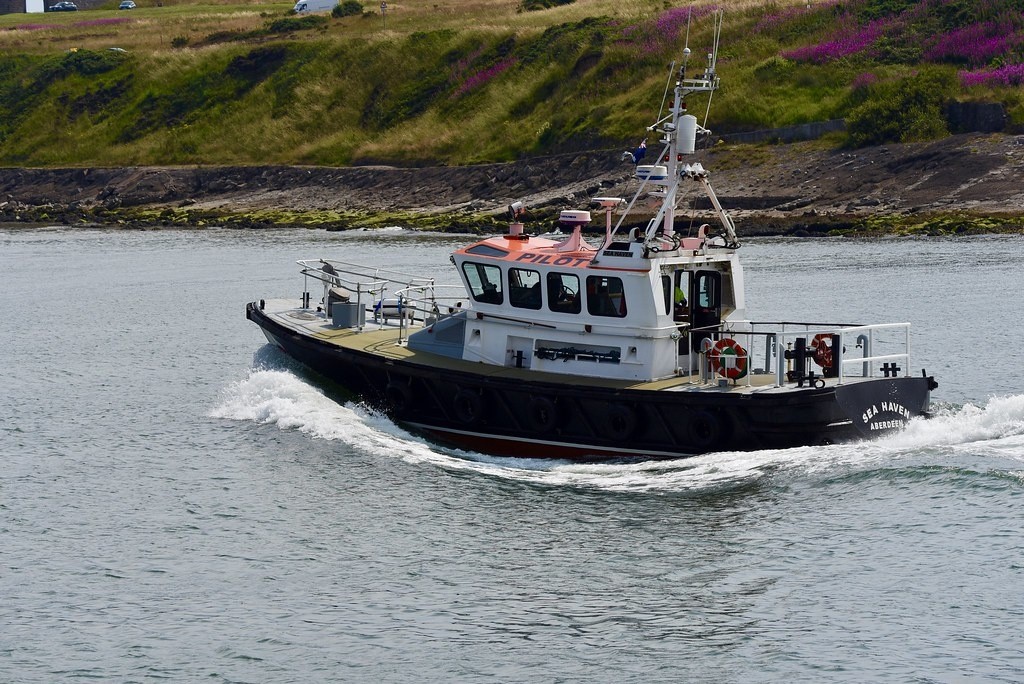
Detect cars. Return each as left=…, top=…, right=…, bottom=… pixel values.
left=119, top=0, right=138, bottom=10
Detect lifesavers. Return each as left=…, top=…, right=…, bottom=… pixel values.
left=810, top=333, right=837, bottom=368
left=712, top=338, right=746, bottom=378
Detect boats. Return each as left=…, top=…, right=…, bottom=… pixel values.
left=373, top=298, right=416, bottom=317
left=246, top=3, right=942, bottom=459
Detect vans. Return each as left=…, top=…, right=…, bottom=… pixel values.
left=293, top=0, right=339, bottom=12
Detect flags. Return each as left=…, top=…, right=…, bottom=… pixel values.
left=620, top=138, right=648, bottom=167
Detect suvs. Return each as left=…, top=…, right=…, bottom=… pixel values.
left=48, top=1, right=77, bottom=11
